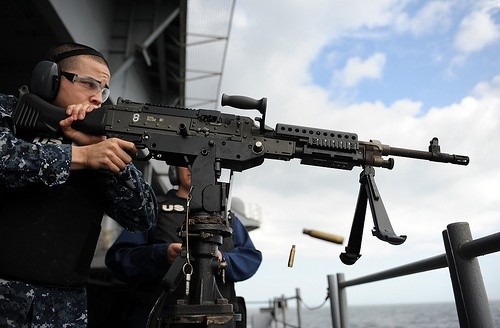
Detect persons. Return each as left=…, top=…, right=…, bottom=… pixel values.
left=105, top=166, right=263, bottom=328
left=0, top=42, right=159, bottom=328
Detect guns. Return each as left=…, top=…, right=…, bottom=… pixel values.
left=10, top=93, right=469, bottom=265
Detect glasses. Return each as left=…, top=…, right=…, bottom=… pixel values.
left=59, top=71, right=110, bottom=103
